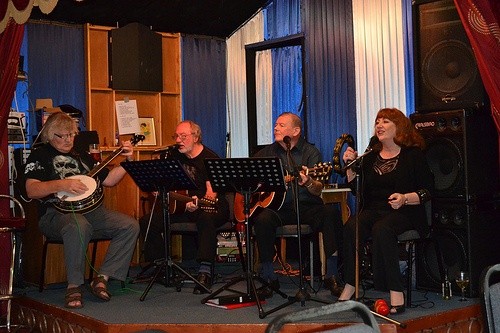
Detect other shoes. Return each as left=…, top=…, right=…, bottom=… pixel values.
left=256, top=278, right=281, bottom=298
left=137, top=266, right=171, bottom=281
left=193, top=271, right=211, bottom=295
left=336, top=282, right=355, bottom=302
left=325, top=272, right=344, bottom=296
left=389, top=291, right=405, bottom=314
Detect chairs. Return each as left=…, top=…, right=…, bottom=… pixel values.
left=266, top=300, right=381, bottom=333
left=368, top=170, right=446, bottom=309
left=168, top=193, right=246, bottom=286
left=478, top=264, right=500, bottom=333
left=35, top=130, right=112, bottom=293
left=251, top=143, right=317, bottom=293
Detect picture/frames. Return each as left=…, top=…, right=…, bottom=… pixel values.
left=135, top=118, right=157, bottom=146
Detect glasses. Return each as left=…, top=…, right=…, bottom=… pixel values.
left=172, top=133, right=195, bottom=140
left=54, top=132, right=76, bottom=139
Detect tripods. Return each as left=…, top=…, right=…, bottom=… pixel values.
left=120, top=157, right=334, bottom=318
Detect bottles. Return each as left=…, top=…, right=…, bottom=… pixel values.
left=441, top=268, right=452, bottom=300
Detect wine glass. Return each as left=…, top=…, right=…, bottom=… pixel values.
left=455, top=271, right=469, bottom=302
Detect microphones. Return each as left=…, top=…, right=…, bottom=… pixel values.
left=283, top=136, right=291, bottom=149
left=370, top=144, right=382, bottom=153
left=154, top=144, right=179, bottom=152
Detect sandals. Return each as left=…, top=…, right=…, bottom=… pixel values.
left=88, top=275, right=111, bottom=301
left=65, top=287, right=84, bottom=309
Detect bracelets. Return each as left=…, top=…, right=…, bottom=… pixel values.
left=404, top=198, right=408, bottom=205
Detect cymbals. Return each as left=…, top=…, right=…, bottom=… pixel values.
left=332, top=133, right=355, bottom=174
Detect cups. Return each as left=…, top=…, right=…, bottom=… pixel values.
left=89, top=144, right=101, bottom=163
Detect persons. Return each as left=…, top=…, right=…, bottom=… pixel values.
left=24, top=112, right=140, bottom=309
left=137, top=121, right=230, bottom=295
left=337, top=109, right=436, bottom=316
left=253, top=112, right=344, bottom=297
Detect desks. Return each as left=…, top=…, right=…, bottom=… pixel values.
left=319, top=188, right=352, bottom=280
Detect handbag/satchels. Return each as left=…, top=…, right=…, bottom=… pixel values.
left=57, top=104, right=86, bottom=128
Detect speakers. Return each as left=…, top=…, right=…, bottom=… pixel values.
left=112, top=23, right=164, bottom=92
left=408, top=0, right=500, bottom=298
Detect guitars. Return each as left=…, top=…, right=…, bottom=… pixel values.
left=232, top=161, right=333, bottom=223
left=51, top=134, right=145, bottom=216
left=148, top=189, right=220, bottom=215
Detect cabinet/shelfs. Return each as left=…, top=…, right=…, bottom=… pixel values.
left=83, top=23, right=183, bottom=264
left=7, top=75, right=27, bottom=143
